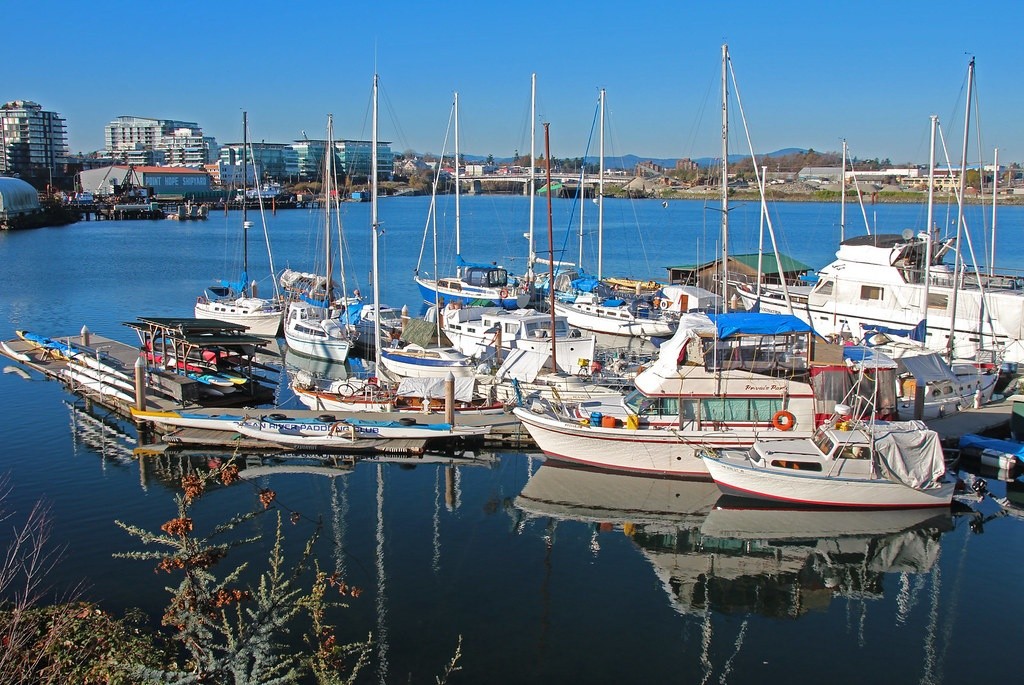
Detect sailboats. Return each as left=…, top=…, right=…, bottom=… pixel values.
left=286, top=45, right=1024, bottom=441
left=194, top=109, right=285, bottom=339
left=736, top=54, right=1023, bottom=365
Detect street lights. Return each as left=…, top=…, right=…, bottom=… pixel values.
left=48, top=164, right=54, bottom=192
left=73, top=173, right=80, bottom=191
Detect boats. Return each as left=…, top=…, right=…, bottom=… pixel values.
left=129, top=406, right=533, bottom=445
left=512, top=368, right=817, bottom=479
left=699, top=418, right=957, bottom=509
left=71, top=191, right=94, bottom=205
left=700, top=495, right=948, bottom=579
left=513, top=458, right=881, bottom=621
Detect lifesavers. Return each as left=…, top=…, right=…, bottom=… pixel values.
left=319, top=413, right=336, bottom=422
left=398, top=417, right=417, bottom=425
left=772, top=410, right=794, bottom=432
left=269, top=413, right=286, bottom=420
left=499, top=290, right=509, bottom=299
left=660, top=300, right=668, bottom=310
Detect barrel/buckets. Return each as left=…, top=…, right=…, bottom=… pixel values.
left=590, top=412, right=602, bottom=426
left=602, top=416, right=615, bottom=428
left=627, top=415, right=638, bottom=430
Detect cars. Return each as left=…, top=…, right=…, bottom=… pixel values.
left=820, top=177, right=834, bottom=185
left=797, top=177, right=806, bottom=183
left=776, top=180, right=785, bottom=184
left=770, top=179, right=778, bottom=185
left=806, top=176, right=820, bottom=183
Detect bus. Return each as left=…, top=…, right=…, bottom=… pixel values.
left=900, top=178, right=961, bottom=190
